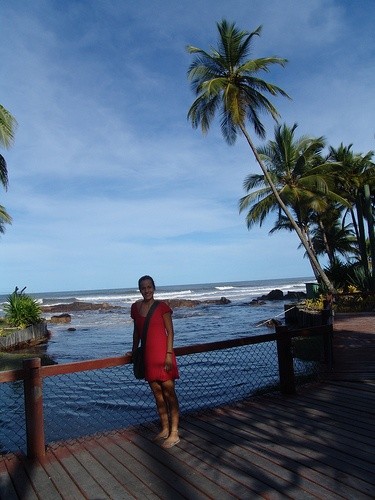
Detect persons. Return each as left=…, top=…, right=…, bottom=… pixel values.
left=131, top=275, right=181, bottom=449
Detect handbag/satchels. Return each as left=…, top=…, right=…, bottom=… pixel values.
left=134, top=349, right=144, bottom=379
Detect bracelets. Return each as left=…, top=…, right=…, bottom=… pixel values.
left=167, top=351, right=172, bottom=354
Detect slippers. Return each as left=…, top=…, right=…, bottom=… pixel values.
left=161, top=431, right=180, bottom=448
left=151, top=432, right=170, bottom=441
